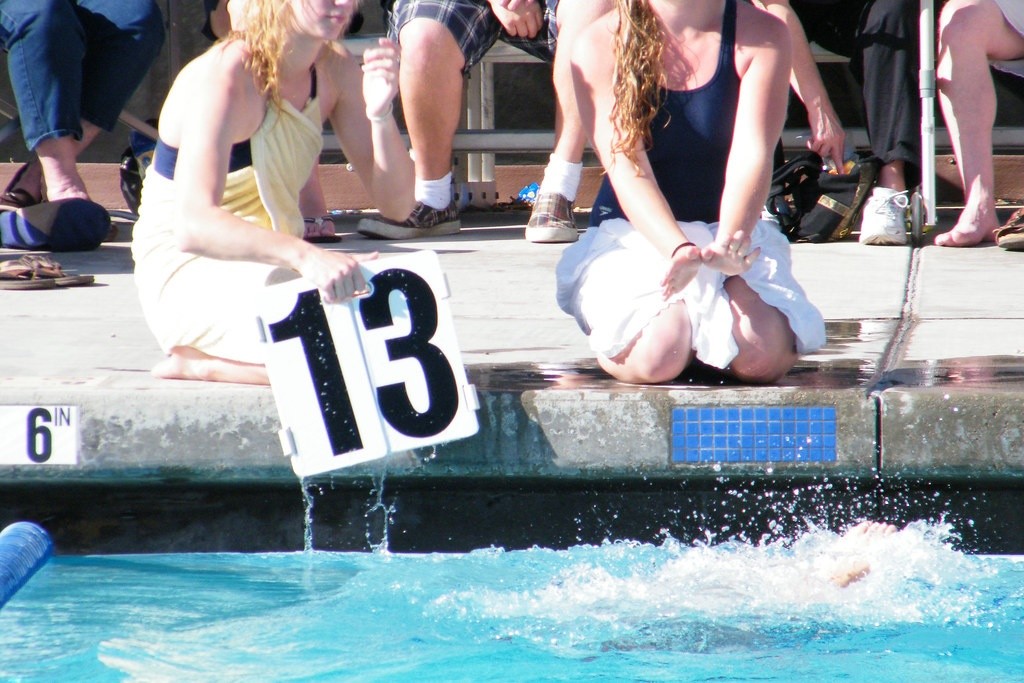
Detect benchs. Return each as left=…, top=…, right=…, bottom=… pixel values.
left=316, top=36, right=1024, bottom=212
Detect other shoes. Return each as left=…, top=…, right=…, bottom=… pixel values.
left=525, top=190, right=580, bottom=244
left=357, top=196, right=463, bottom=240
left=0, top=161, right=42, bottom=211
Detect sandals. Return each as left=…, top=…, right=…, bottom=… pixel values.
left=993, top=205, right=1024, bottom=250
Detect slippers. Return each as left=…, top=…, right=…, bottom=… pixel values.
left=303, top=216, right=342, bottom=245
left=37, top=254, right=96, bottom=287
left=0, top=258, right=60, bottom=291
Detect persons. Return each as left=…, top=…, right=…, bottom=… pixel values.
left=357, top=0, right=624, bottom=244
left=130, top=0, right=415, bottom=385
left=936, top=0, right=1024, bottom=246
left=555, top=0, right=825, bottom=383
left=202, top=0, right=344, bottom=243
left=750, top=0, right=916, bottom=242
left=0, top=0, right=165, bottom=212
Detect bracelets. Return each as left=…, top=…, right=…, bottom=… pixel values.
left=671, top=242, right=696, bottom=258
left=365, top=103, right=393, bottom=121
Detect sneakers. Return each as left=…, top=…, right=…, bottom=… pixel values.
left=858, top=189, right=909, bottom=245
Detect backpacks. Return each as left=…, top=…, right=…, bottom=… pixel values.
left=766, top=149, right=870, bottom=245
